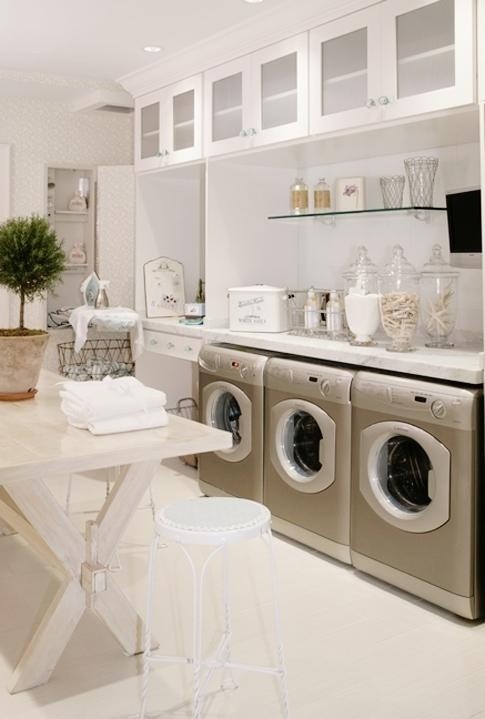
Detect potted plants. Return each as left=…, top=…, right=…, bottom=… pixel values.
left=0, top=210, right=67, bottom=401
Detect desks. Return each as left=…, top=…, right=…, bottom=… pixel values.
left=0, top=367, right=236, bottom=698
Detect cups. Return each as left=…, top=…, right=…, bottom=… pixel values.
left=380, top=175, right=405, bottom=210
left=403, top=156, right=441, bottom=208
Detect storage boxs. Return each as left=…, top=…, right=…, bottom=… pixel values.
left=227, top=284, right=296, bottom=335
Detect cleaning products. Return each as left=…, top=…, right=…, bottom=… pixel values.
left=304, top=286, right=319, bottom=331
left=95, top=279, right=110, bottom=310
left=325, top=290, right=342, bottom=334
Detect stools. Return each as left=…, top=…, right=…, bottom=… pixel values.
left=136, top=491, right=297, bottom=719
left=61, top=463, right=157, bottom=532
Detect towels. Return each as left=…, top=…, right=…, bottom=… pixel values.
left=59, top=373, right=170, bottom=438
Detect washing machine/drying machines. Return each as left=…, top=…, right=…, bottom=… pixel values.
left=198, top=343, right=262, bottom=511
left=264, top=360, right=352, bottom=568
left=350, top=371, right=480, bottom=619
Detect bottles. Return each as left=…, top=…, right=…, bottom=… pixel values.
left=68, top=189, right=87, bottom=210
left=304, top=287, right=341, bottom=333
left=69, top=241, right=86, bottom=264
left=341, top=244, right=460, bottom=352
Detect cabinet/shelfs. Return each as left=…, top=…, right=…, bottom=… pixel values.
left=45, top=163, right=97, bottom=330
left=204, top=30, right=310, bottom=160
left=133, top=72, right=202, bottom=174
left=309, top=0, right=476, bottom=138
